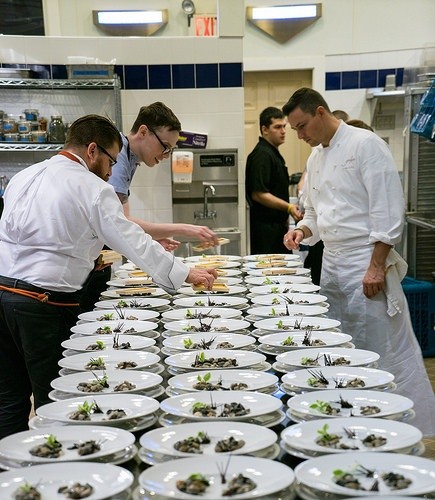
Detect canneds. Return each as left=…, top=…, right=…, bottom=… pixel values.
left=0, top=109, right=48, bottom=142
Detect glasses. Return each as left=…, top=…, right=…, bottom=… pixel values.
left=86, top=144, right=117, bottom=166
left=139, top=122, right=172, bottom=154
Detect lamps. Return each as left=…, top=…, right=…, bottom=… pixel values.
left=182, top=0, right=217, bottom=36
left=92, top=9, right=168, bottom=36
left=245, top=3, right=323, bottom=46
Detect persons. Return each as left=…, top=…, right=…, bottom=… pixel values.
left=108, top=101, right=216, bottom=251
left=0, top=115, right=218, bottom=444
left=244, top=107, right=303, bottom=255
left=282, top=88, right=435, bottom=438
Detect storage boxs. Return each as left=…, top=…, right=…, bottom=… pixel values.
left=399, top=275, right=435, bottom=359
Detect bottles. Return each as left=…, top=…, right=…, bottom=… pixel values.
left=0, top=108, right=72, bottom=143
left=0, top=175, right=9, bottom=196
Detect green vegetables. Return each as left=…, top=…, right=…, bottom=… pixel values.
left=17, top=276, right=348, bottom=496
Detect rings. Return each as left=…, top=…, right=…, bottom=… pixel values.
left=285, top=239, right=288, bottom=241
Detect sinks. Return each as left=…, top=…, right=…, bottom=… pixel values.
left=173, top=227, right=241, bottom=243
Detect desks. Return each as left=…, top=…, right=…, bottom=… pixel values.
left=109, top=272, right=310, bottom=500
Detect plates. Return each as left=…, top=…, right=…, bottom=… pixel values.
left=0, top=252, right=435, bottom=500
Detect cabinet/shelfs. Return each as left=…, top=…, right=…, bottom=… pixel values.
left=0, top=73, right=124, bottom=152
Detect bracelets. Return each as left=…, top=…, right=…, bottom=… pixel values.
left=287, top=203, right=293, bottom=214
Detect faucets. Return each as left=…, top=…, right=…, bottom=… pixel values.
left=193, top=185, right=217, bottom=220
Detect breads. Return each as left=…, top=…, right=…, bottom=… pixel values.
left=94, top=238, right=296, bottom=295
